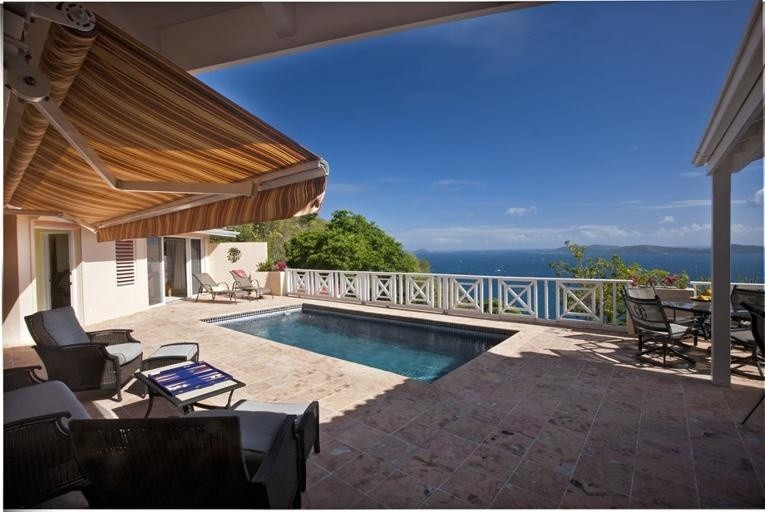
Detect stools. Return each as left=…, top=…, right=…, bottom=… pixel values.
left=230, top=398, right=320, bottom=492
left=140, top=342, right=199, bottom=372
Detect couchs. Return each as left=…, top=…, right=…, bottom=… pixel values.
left=4, top=365, right=92, bottom=508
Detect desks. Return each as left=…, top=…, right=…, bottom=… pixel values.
left=134, top=360, right=246, bottom=419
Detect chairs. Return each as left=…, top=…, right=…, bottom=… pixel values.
left=193, top=272, right=237, bottom=304
left=620, top=278, right=765, bottom=426
left=228, top=269, right=273, bottom=301
left=67, top=414, right=302, bottom=508
left=24, top=305, right=143, bottom=401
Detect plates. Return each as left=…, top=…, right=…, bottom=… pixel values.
left=689, top=299, right=713, bottom=303
